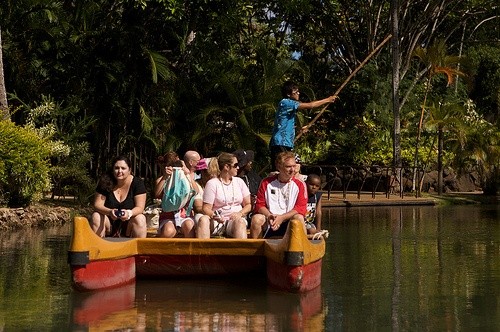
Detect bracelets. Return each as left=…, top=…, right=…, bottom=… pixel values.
left=127, top=210, right=133, bottom=219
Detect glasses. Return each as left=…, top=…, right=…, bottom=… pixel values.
left=291, top=90, right=299, bottom=96
left=229, top=162, right=240, bottom=168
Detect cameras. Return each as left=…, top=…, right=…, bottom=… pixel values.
left=115, top=211, right=125, bottom=217
left=215, top=210, right=222, bottom=218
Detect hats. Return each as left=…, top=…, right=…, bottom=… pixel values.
left=235, top=149, right=254, bottom=167
left=194, top=158, right=213, bottom=176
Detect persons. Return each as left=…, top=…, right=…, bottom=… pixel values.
left=269, top=82, right=337, bottom=171
left=94, top=150, right=323, bottom=239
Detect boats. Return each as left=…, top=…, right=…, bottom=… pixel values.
left=67, top=215, right=325, bottom=293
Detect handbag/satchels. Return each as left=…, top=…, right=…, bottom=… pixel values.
left=161, top=166, right=191, bottom=212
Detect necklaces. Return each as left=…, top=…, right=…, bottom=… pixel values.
left=220, top=176, right=235, bottom=207
left=277, top=175, right=289, bottom=210
